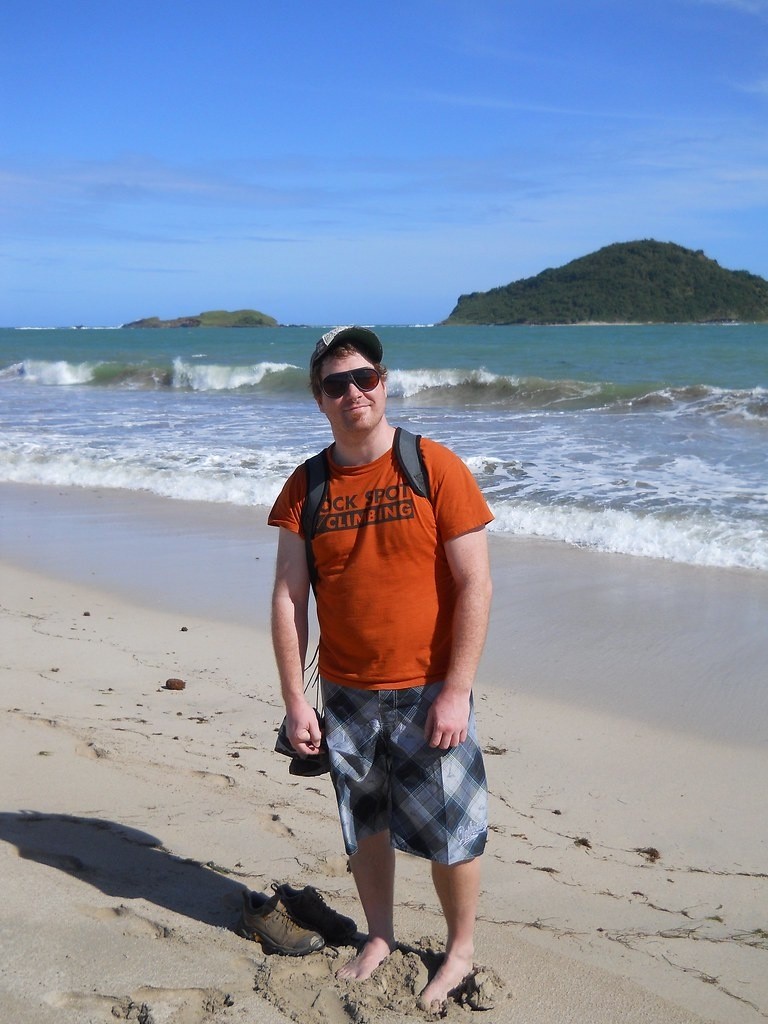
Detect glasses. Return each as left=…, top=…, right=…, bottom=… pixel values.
left=319, top=367, right=387, bottom=399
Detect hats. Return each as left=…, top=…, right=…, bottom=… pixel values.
left=310, top=325, right=383, bottom=372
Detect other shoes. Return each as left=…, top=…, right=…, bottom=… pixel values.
left=275, top=708, right=330, bottom=777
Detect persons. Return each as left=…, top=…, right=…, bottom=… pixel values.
left=267, top=325, right=495, bottom=1014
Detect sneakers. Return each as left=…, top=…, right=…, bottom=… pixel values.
left=236, top=883, right=357, bottom=957
